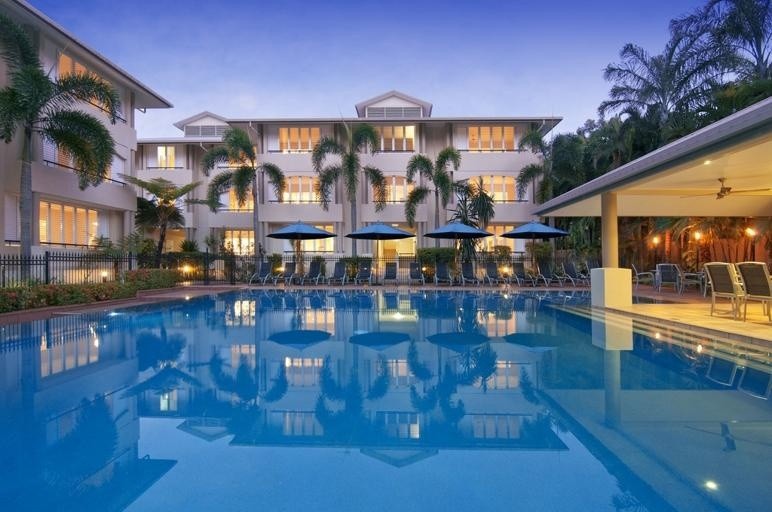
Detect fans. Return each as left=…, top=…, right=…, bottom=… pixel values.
left=680, top=178, right=772, bottom=200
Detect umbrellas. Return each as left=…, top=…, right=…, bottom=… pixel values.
left=265, top=219, right=338, bottom=285
left=425, top=331, right=492, bottom=354
left=268, top=295, right=332, bottom=351
left=349, top=291, right=409, bottom=351
left=503, top=313, right=561, bottom=353
left=499, top=220, right=571, bottom=266
left=424, top=221, right=494, bottom=262
left=343, top=221, right=417, bottom=286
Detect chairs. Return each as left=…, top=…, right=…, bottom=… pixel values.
left=631, top=261, right=771, bottom=327
left=382, top=261, right=398, bottom=286
left=510, top=262, right=536, bottom=287
left=408, top=263, right=425, bottom=287
left=249, top=260, right=273, bottom=287
left=483, top=262, right=508, bottom=286
left=353, top=261, right=373, bottom=286
left=561, top=262, right=589, bottom=287
left=272, top=262, right=301, bottom=287
left=458, top=262, right=479, bottom=287
left=433, top=260, right=452, bottom=287
left=535, top=260, right=563, bottom=287
left=327, top=261, right=349, bottom=286
left=585, top=261, right=598, bottom=277
left=300, top=260, right=324, bottom=287
